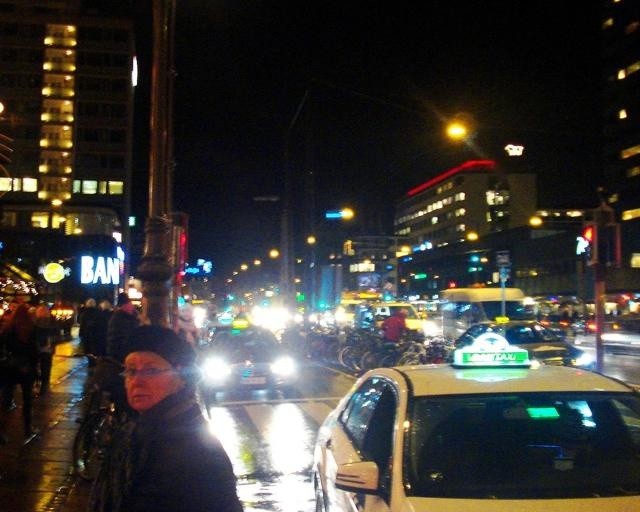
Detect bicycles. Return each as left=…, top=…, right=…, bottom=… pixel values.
left=70, top=352, right=122, bottom=482
left=280, top=320, right=448, bottom=373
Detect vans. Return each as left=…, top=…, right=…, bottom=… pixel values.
left=444, top=288, right=534, bottom=328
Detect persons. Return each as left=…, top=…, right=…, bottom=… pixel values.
left=381, top=308, right=410, bottom=343
left=1, top=301, right=56, bottom=444
left=75, top=291, right=146, bottom=409
left=86, top=325, right=245, bottom=512
left=180, top=303, right=201, bottom=349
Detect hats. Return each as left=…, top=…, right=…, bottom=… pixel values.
left=123, top=324, right=197, bottom=379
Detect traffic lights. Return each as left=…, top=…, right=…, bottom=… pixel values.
left=580, top=226, right=593, bottom=260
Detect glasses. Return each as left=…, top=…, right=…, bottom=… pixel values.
left=124, top=367, right=174, bottom=377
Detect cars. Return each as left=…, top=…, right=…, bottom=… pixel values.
left=314, top=334, right=639, bottom=511
left=194, top=321, right=289, bottom=396
left=538, top=295, right=640, bottom=353
left=341, top=299, right=427, bottom=335
left=454, top=318, right=575, bottom=368
